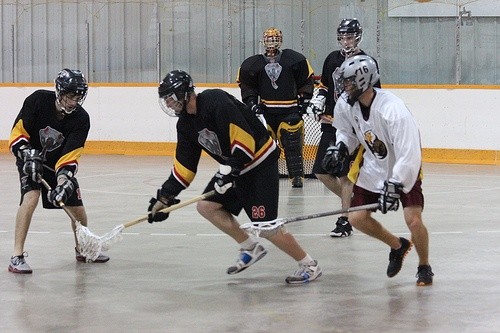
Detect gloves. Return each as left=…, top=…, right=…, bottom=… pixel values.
left=321, top=141, right=349, bottom=175
left=297, top=96, right=310, bottom=117
left=212, top=155, right=243, bottom=196
left=250, top=102, right=265, bottom=115
left=47, top=171, right=75, bottom=208
left=21, top=149, right=44, bottom=184
left=147, top=189, right=181, bottom=223
left=377, top=180, right=404, bottom=215
left=312, top=96, right=326, bottom=122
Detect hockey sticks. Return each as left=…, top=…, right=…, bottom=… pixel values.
left=319, top=115, right=332, bottom=122
left=37, top=173, right=104, bottom=263
left=98, top=190, right=215, bottom=251
left=239, top=202, right=381, bottom=241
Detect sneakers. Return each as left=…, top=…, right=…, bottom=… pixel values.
left=386, top=237, right=413, bottom=278
left=227, top=242, right=266, bottom=275
left=8, top=252, right=33, bottom=274
left=286, top=260, right=322, bottom=284
left=416, top=266, right=434, bottom=286
left=329, top=217, right=353, bottom=237
left=75, top=246, right=109, bottom=262
left=292, top=176, right=303, bottom=188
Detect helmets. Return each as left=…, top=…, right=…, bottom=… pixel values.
left=158, top=70, right=193, bottom=117
left=335, top=55, right=381, bottom=108
left=55, top=69, right=88, bottom=116
left=264, top=28, right=283, bottom=56
left=337, top=19, right=362, bottom=53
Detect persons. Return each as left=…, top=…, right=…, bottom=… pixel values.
left=310, top=18, right=381, bottom=238
left=8, top=68, right=110, bottom=274
left=237, top=27, right=314, bottom=188
left=323, top=55, right=435, bottom=286
left=148, top=70, right=322, bottom=284
